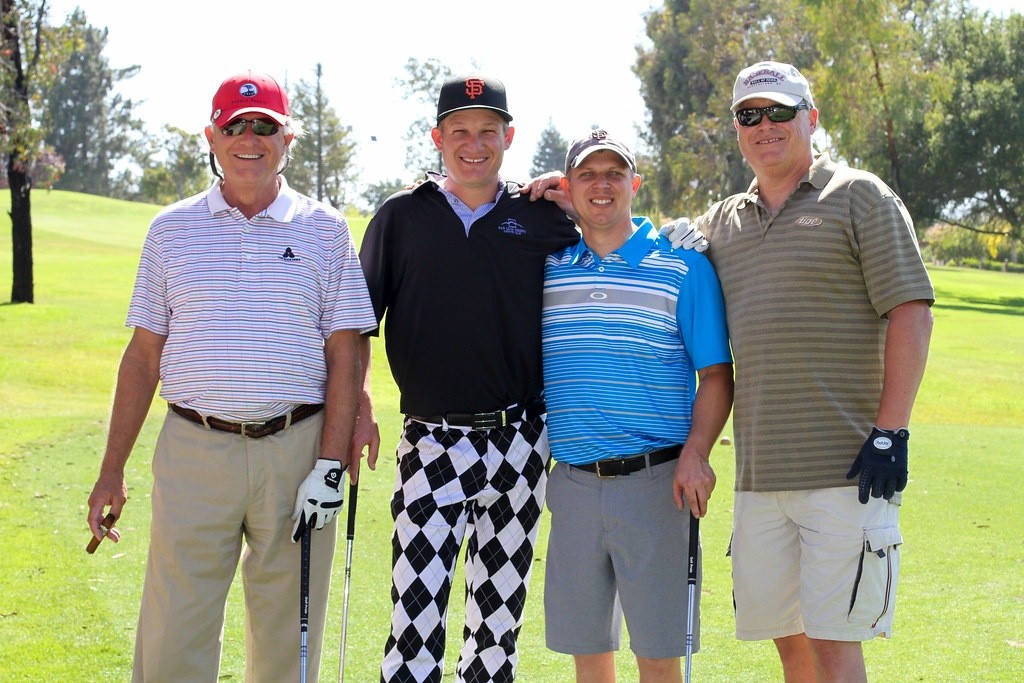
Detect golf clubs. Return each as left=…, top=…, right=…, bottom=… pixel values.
left=685, top=506, right=699, bottom=683
left=337, top=462, right=360, bottom=682
left=300, top=520, right=312, bottom=682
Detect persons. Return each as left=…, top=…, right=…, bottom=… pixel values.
left=521, top=60, right=935, bottom=683
left=348, top=73, right=710, bottom=683
left=86, top=71, right=377, bottom=683
left=406, top=128, right=734, bottom=683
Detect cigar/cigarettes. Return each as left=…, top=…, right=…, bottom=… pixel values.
left=86, top=515, right=115, bottom=554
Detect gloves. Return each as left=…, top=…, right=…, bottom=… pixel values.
left=659, top=217, right=709, bottom=252
left=291, top=458, right=349, bottom=543
left=846, top=427, right=909, bottom=504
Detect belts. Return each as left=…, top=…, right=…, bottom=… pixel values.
left=410, top=403, right=535, bottom=429
left=569, top=444, right=684, bottom=479
left=169, top=403, right=324, bottom=438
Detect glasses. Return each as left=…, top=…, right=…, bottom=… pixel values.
left=221, top=118, right=284, bottom=136
left=735, top=104, right=808, bottom=127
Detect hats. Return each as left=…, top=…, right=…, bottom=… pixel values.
left=437, top=75, right=513, bottom=127
left=565, top=129, right=637, bottom=176
left=210, top=71, right=291, bottom=126
left=731, top=62, right=814, bottom=112
